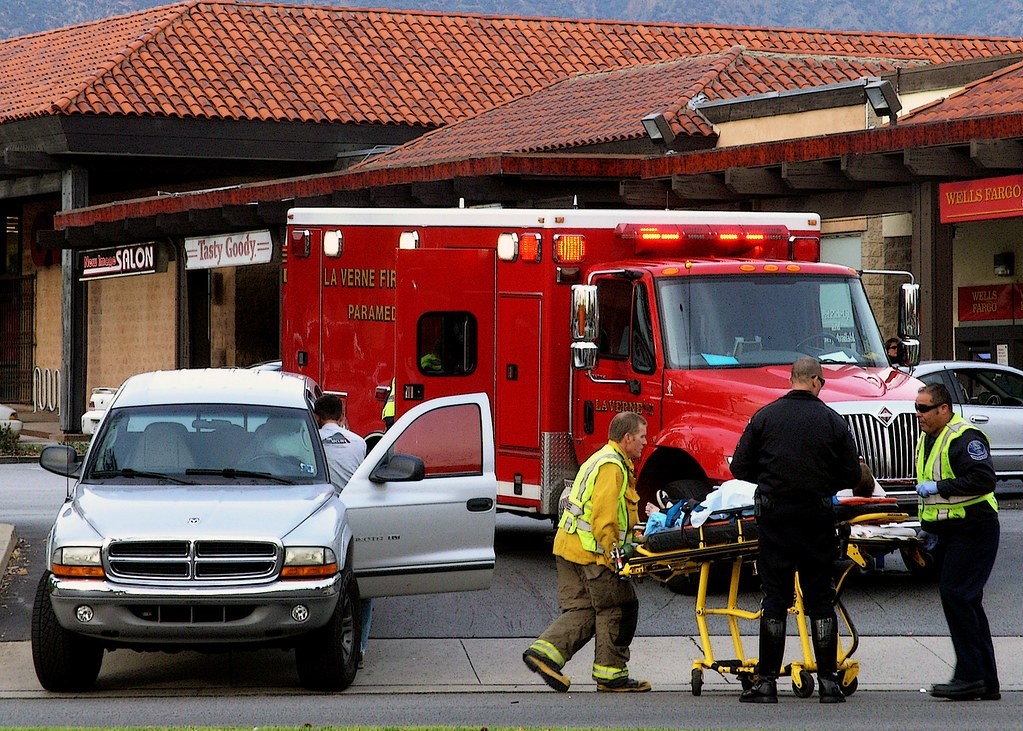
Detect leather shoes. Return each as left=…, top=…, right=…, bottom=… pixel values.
left=929, top=678, right=1001, bottom=701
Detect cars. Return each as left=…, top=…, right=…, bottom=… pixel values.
left=904, top=359, right=1023, bottom=483
left=0, top=403, right=23, bottom=440
left=247, top=360, right=283, bottom=372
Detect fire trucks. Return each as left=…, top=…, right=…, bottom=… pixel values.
left=279, top=205, right=937, bottom=596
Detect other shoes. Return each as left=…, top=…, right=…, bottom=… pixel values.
left=657, top=490, right=671, bottom=508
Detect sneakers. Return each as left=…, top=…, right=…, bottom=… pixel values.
left=739, top=679, right=778, bottom=703
left=597, top=679, right=651, bottom=692
left=817, top=675, right=847, bottom=703
left=523, top=654, right=571, bottom=693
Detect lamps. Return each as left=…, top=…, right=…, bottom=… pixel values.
left=641, top=113, right=679, bottom=154
left=864, top=81, right=904, bottom=126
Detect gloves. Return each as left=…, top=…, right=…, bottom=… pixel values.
left=917, top=530, right=938, bottom=552
left=915, top=481, right=937, bottom=499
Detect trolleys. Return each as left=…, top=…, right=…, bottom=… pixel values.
left=608, top=512, right=934, bottom=698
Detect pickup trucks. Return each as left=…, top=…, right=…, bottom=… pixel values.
left=81, top=386, right=118, bottom=439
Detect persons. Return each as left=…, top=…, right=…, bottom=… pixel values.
left=382, top=336, right=445, bottom=435
left=886, top=338, right=899, bottom=364
left=262, top=393, right=374, bottom=670
left=523, top=411, right=653, bottom=694
left=645, top=462, right=875, bottom=531
left=914, top=382, right=1001, bottom=702
left=729, top=357, right=863, bottom=703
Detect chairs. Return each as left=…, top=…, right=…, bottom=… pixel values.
left=124, top=421, right=197, bottom=473
left=237, top=424, right=296, bottom=465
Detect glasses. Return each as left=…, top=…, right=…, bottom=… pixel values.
left=890, top=346, right=897, bottom=349
left=811, top=374, right=825, bottom=388
left=914, top=401, right=946, bottom=413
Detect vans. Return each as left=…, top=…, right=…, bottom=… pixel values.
left=31, top=366, right=497, bottom=693
